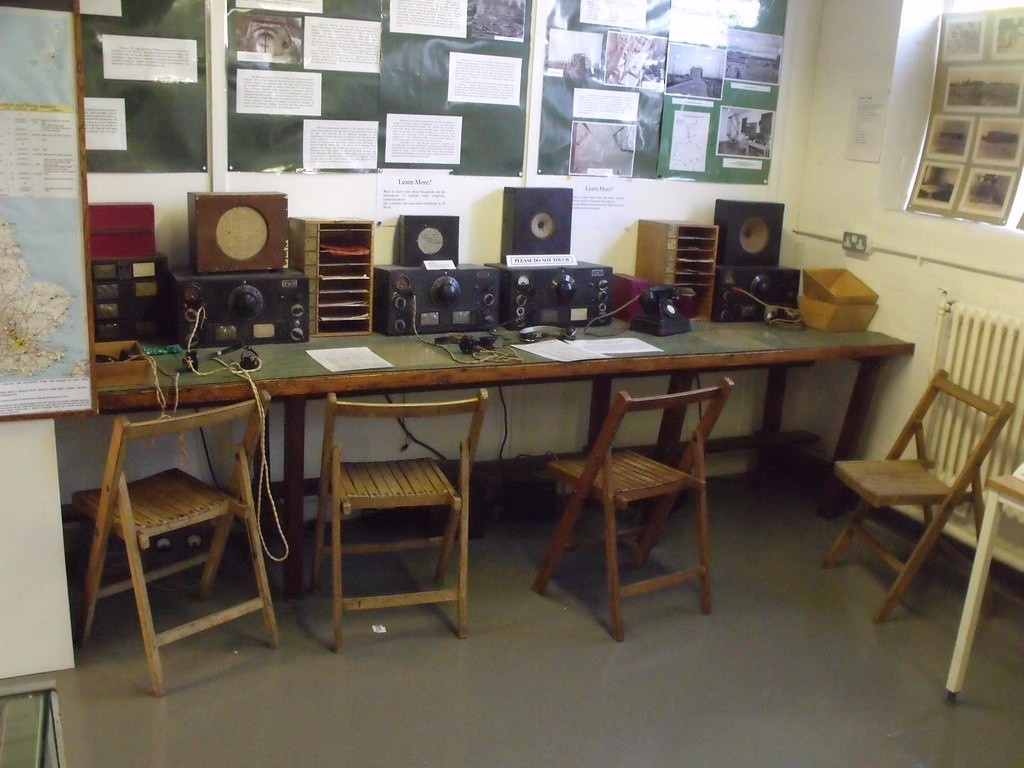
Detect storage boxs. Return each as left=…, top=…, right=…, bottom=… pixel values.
left=287, top=216, right=376, bottom=336
left=610, top=273, right=651, bottom=324
left=88, top=201, right=156, bottom=259
left=797, top=268, right=880, bottom=334
left=634, top=218, right=720, bottom=324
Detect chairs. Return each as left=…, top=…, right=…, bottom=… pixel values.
left=308, top=387, right=492, bottom=654
left=72, top=388, right=282, bottom=698
left=530, top=375, right=736, bottom=646
left=821, top=370, right=1017, bottom=626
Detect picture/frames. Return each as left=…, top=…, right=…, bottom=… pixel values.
left=904, top=6, right=1024, bottom=226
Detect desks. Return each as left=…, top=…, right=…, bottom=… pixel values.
left=943, top=476, right=1024, bottom=707
left=94, top=319, right=916, bottom=522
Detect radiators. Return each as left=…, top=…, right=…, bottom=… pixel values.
left=923, top=286, right=1024, bottom=518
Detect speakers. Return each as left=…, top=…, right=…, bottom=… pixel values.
left=187, top=191, right=288, bottom=273
left=501, top=187, right=573, bottom=263
left=400, top=214, right=459, bottom=266
left=714, top=199, right=785, bottom=266
